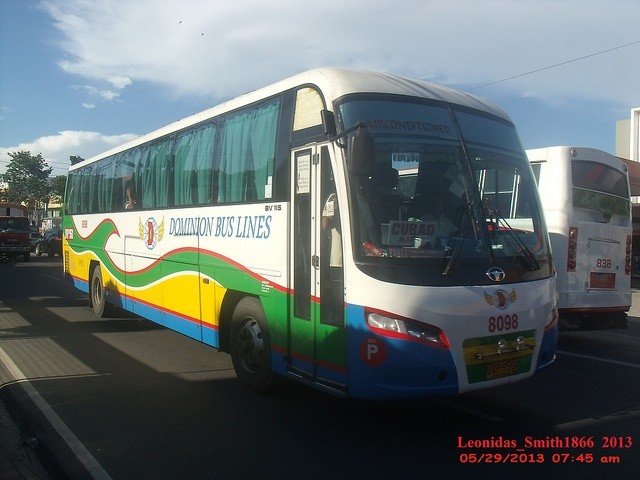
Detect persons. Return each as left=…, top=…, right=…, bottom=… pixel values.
left=125, top=186, right=137, bottom=210
left=323, top=185, right=344, bottom=322
left=420, top=193, right=464, bottom=239
left=382, top=167, right=411, bottom=202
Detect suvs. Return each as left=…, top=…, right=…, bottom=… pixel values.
left=36, top=230, right=62, bottom=258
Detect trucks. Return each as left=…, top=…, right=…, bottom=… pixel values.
left=0, top=216, right=32, bottom=262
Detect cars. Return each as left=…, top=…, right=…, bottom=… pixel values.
left=31, top=231, right=43, bottom=249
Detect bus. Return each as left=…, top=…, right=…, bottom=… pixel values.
left=473, top=144, right=634, bottom=329
left=60, top=64, right=560, bottom=416
